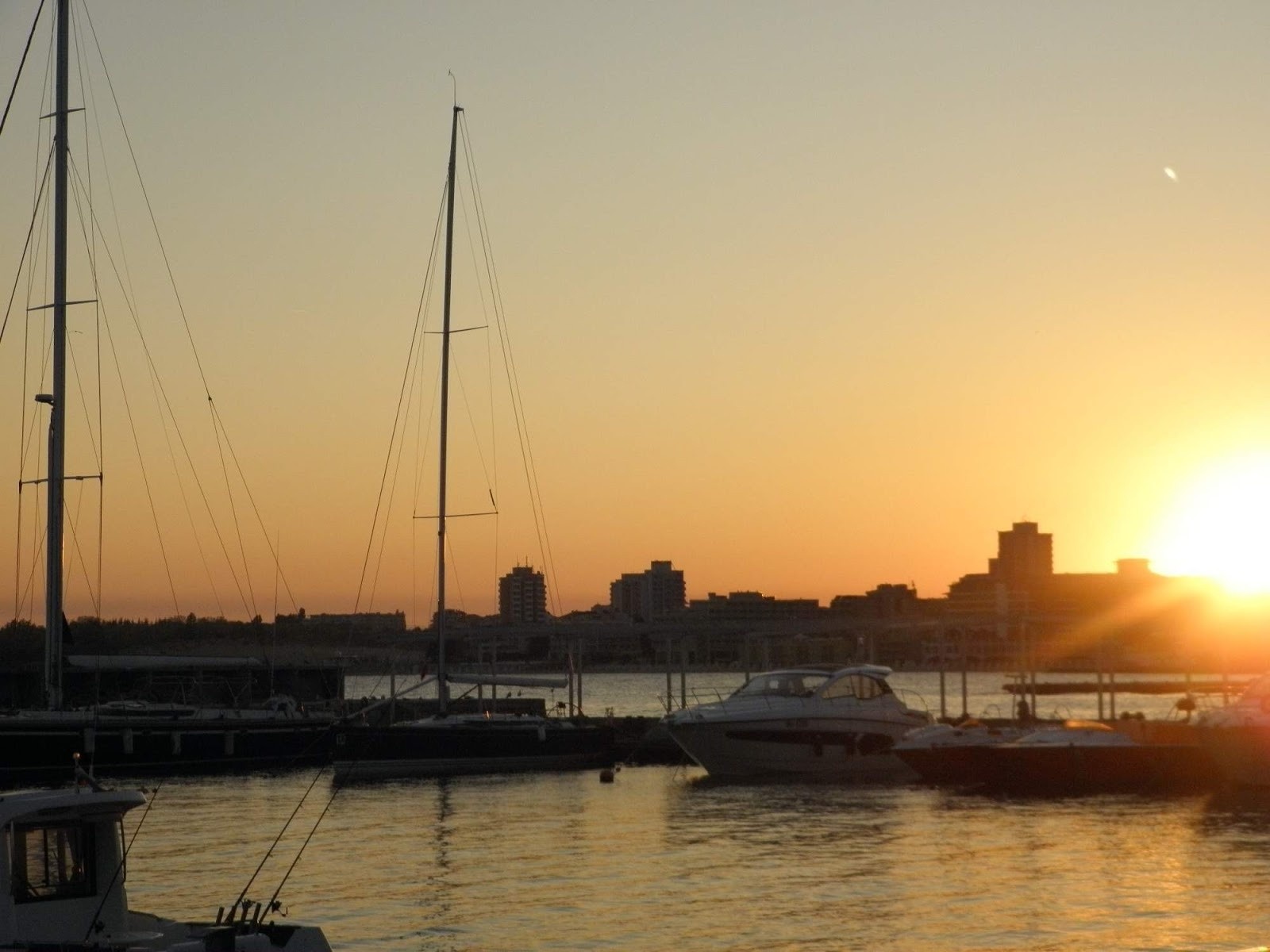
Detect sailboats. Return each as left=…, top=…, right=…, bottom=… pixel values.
left=0, top=0, right=614, bottom=790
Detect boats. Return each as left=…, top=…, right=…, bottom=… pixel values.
left=655, top=626, right=1270, bottom=812
left=0, top=759, right=333, bottom=952
